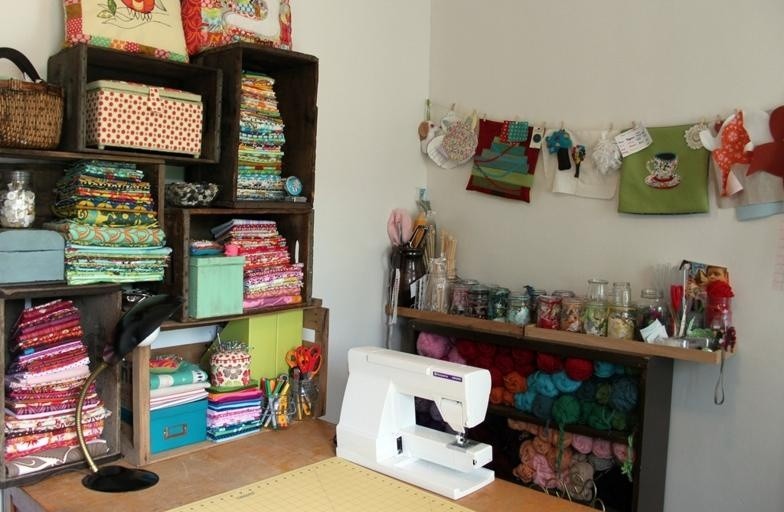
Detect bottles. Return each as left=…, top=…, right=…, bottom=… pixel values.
left=1, top=170, right=37, bottom=226
left=450, top=278, right=669, bottom=348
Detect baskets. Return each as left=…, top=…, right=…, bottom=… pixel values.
left=0, top=78, right=64, bottom=150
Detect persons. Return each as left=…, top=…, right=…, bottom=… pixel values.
left=706, top=266, right=727, bottom=282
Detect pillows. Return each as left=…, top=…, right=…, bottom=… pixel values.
left=61, top=0, right=188, bottom=63
left=180, top=0, right=293, bottom=56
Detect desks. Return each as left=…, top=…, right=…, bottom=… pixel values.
left=2, top=418, right=606, bottom=512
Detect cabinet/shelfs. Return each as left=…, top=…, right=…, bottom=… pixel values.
left=0, top=41, right=322, bottom=484
left=405, top=317, right=673, bottom=512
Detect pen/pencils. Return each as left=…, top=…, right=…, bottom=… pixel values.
left=260, top=374, right=291, bottom=430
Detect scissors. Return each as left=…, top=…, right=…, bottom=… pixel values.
left=286, top=343, right=322, bottom=420
